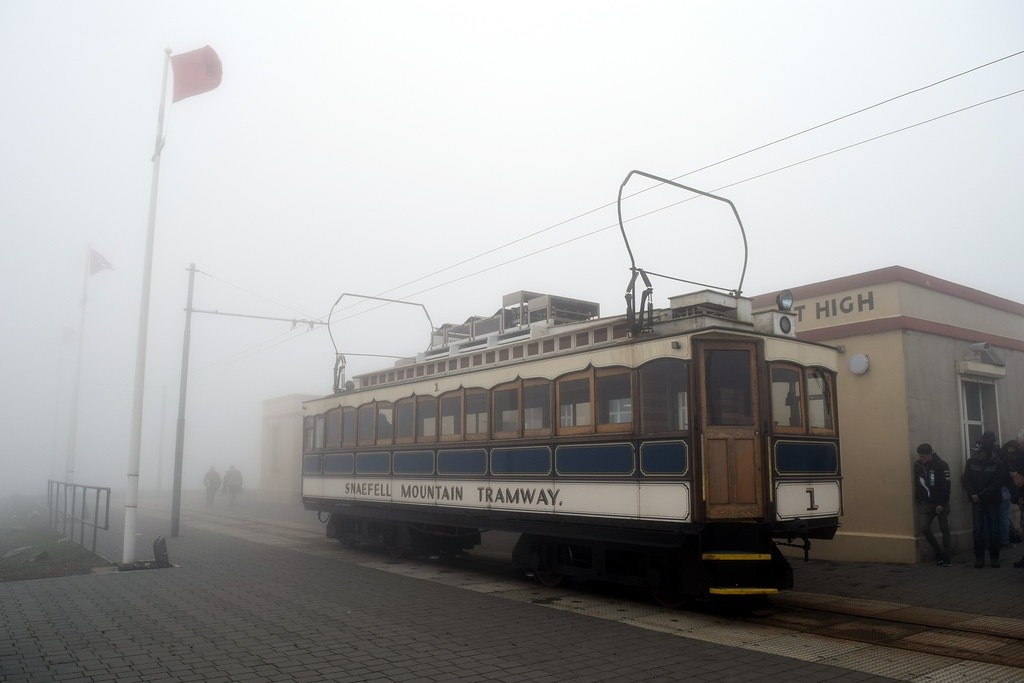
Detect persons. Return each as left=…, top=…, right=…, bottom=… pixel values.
left=221, top=466, right=242, bottom=505
left=203, top=466, right=222, bottom=505
left=980, top=432, right=1010, bottom=546
left=964, top=439, right=1004, bottom=568
left=1005, top=429, right=1024, bottom=566
left=914, top=444, right=955, bottom=566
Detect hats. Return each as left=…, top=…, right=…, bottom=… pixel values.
left=972, top=441, right=992, bottom=453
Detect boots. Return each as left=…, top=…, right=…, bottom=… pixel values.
left=974, top=544, right=1001, bottom=569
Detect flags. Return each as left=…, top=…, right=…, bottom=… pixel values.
left=169, top=44, right=221, bottom=104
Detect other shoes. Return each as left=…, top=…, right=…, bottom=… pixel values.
left=936, top=554, right=951, bottom=566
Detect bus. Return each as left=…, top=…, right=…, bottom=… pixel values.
left=302, top=170, right=846, bottom=601
